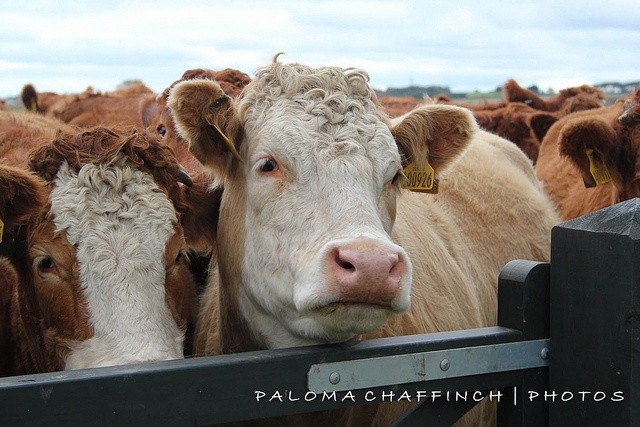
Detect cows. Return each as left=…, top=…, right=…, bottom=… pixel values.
left=0, top=52, right=640, bottom=427
